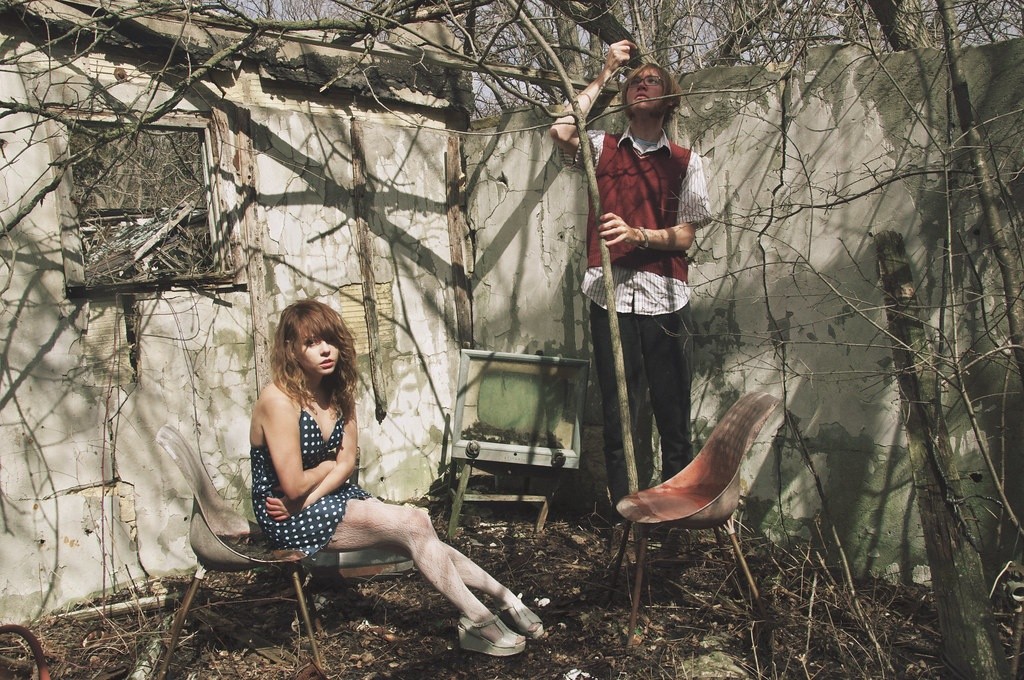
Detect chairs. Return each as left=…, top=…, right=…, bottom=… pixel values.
left=154, top=422, right=331, bottom=680
left=599, top=390, right=784, bottom=652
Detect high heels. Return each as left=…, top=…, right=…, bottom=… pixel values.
left=494, top=592, right=544, bottom=639
left=458, top=613, right=527, bottom=657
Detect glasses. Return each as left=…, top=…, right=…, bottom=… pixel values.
left=625, top=75, right=664, bottom=87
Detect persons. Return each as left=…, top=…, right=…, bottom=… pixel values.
left=248, top=300, right=543, bottom=656
left=550, top=40, right=714, bottom=569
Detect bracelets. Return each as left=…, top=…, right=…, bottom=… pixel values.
left=636, top=225, right=649, bottom=252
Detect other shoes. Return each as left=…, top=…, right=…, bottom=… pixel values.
left=609, top=521, right=640, bottom=569
left=663, top=527, right=692, bottom=559
